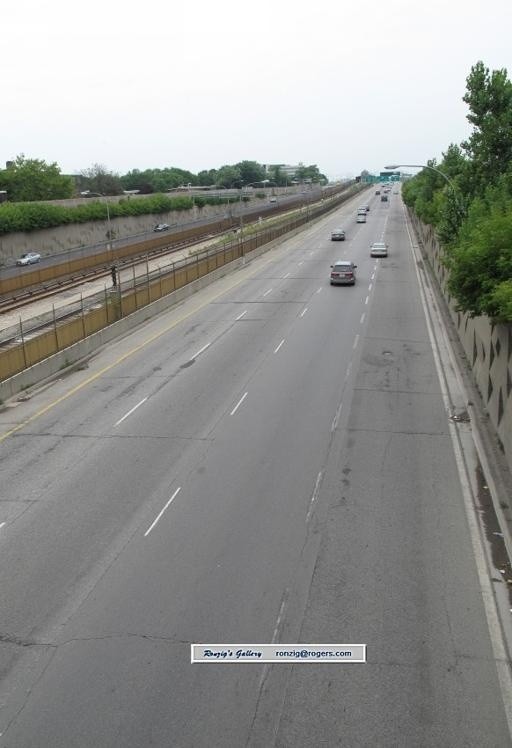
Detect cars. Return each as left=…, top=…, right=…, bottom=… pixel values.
left=270, top=198, right=277, bottom=202
left=16, top=251, right=41, bottom=266
left=370, top=242, right=389, bottom=257
left=154, top=222, right=169, bottom=232
left=331, top=229, right=345, bottom=241
left=372, top=180, right=399, bottom=202
left=357, top=203, right=369, bottom=223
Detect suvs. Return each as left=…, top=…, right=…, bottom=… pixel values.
left=330, top=260, right=358, bottom=286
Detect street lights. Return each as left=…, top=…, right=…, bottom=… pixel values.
left=81, top=190, right=117, bottom=290
left=239, top=179, right=270, bottom=259
left=384, top=164, right=456, bottom=200
